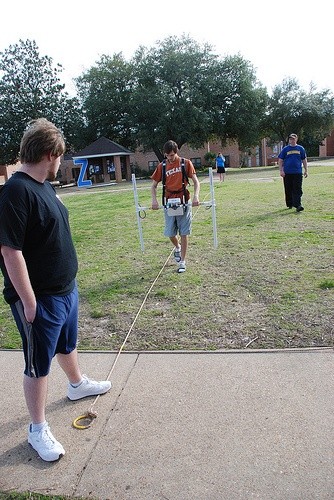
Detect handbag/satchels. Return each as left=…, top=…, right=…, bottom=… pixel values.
left=165, top=202, right=185, bottom=216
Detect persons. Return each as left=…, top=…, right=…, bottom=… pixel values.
left=0, top=117, right=113, bottom=463
left=149, top=140, right=200, bottom=273
left=278, top=134, right=309, bottom=212
left=216, top=152, right=225, bottom=183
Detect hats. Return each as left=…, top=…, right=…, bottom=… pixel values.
left=289, top=134, right=297, bottom=138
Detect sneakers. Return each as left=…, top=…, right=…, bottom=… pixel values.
left=66, top=373, right=112, bottom=400
left=28, top=422, right=66, bottom=463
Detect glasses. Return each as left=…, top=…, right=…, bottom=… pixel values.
left=166, top=153, right=175, bottom=156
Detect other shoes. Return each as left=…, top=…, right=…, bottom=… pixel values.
left=174, top=244, right=182, bottom=262
left=289, top=206, right=292, bottom=209
left=297, top=206, right=303, bottom=212
left=178, top=264, right=185, bottom=273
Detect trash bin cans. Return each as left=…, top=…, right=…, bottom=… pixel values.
left=225, top=154, right=230, bottom=167
